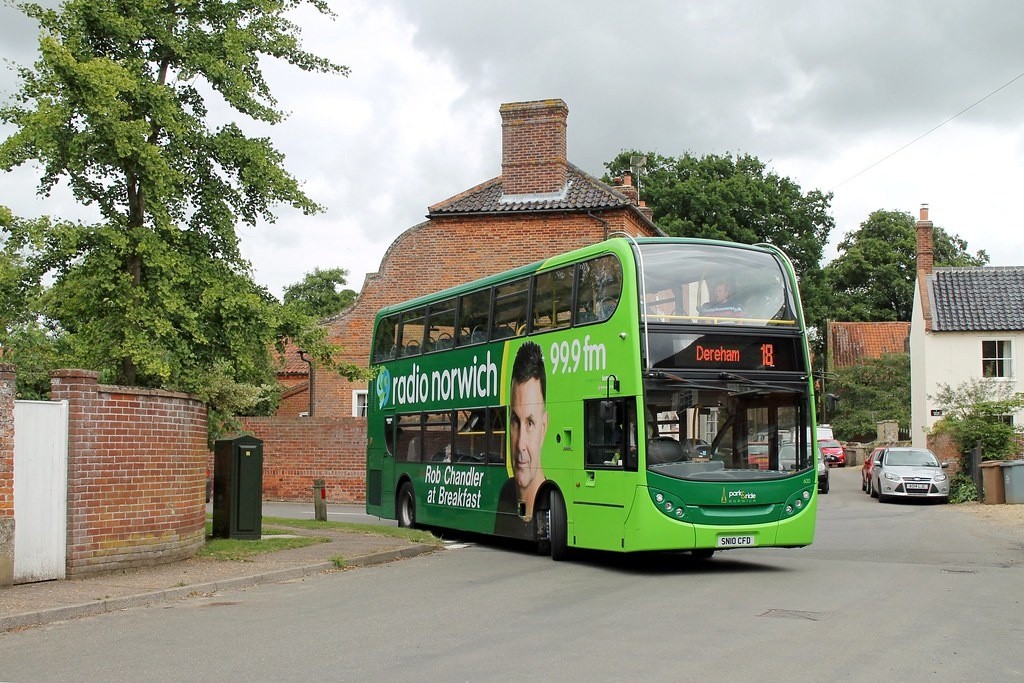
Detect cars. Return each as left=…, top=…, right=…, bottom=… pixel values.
left=205, top=462, right=211, bottom=503
left=861, top=447, right=884, bottom=494
left=870, top=447, right=950, bottom=504
left=778, top=443, right=830, bottom=494
left=817, top=438, right=847, bottom=468
left=747, top=441, right=769, bottom=469
left=719, top=448, right=733, bottom=454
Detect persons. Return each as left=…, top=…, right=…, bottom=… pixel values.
left=494, top=341, right=550, bottom=541
left=699, top=281, right=749, bottom=317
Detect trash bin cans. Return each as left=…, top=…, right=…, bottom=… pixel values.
left=999, top=460, right=1024, bottom=504
left=978, top=460, right=1005, bottom=506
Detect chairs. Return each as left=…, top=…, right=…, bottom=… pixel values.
left=495, top=321, right=515, bottom=341
left=599, top=297, right=617, bottom=320
left=669, top=308, right=690, bottom=322
left=578, top=305, right=597, bottom=325
left=436, top=333, right=453, bottom=351
left=419, top=336, right=435, bottom=354
left=471, top=325, right=488, bottom=345
left=390, top=344, right=405, bottom=359
left=404, top=340, right=419, bottom=357
left=459, top=329, right=471, bottom=346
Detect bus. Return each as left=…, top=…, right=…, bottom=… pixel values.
left=365, top=232, right=818, bottom=567
